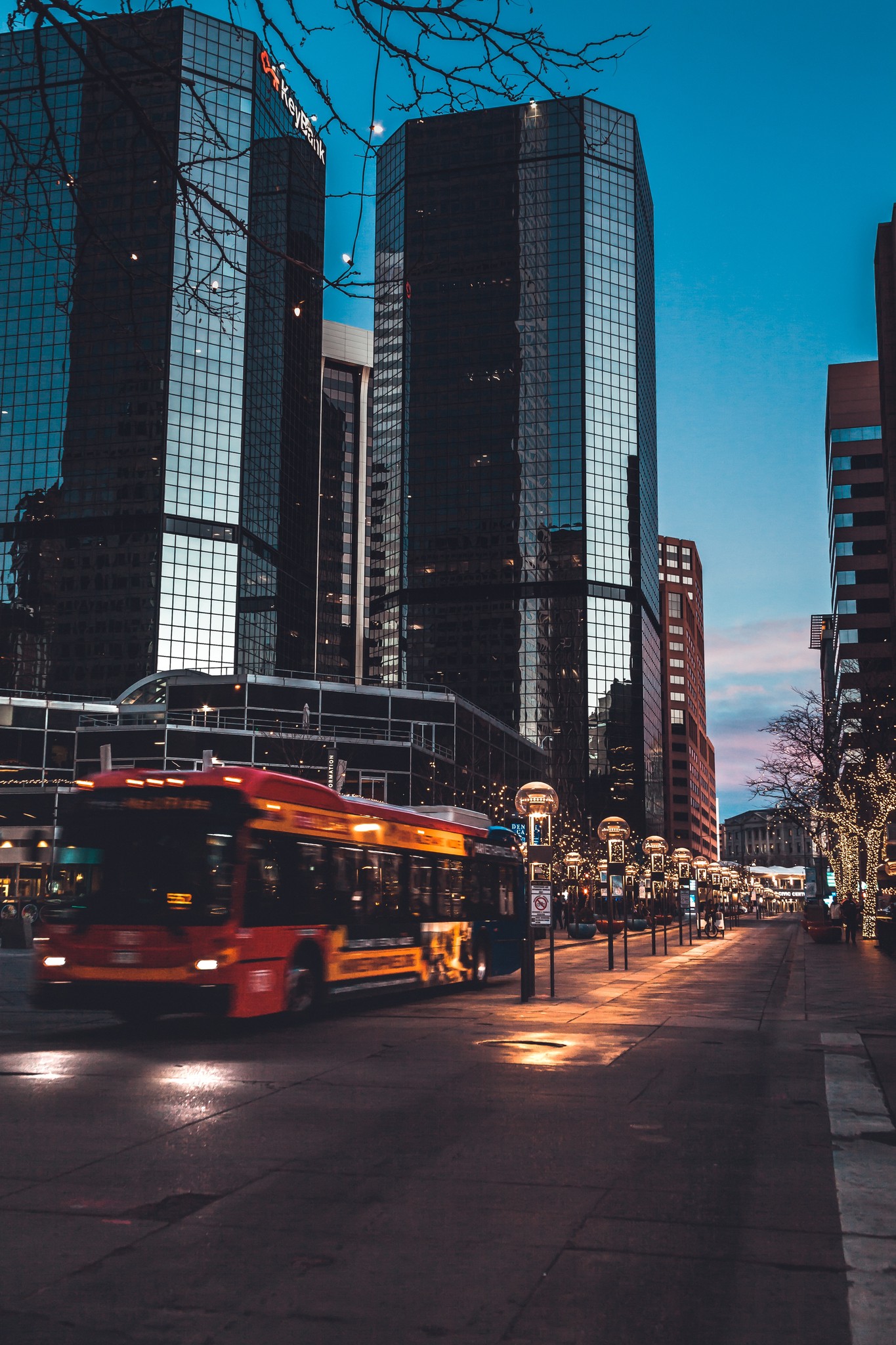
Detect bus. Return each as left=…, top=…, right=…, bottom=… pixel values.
left=32, top=763, right=531, bottom=1019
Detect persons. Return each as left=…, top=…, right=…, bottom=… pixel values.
left=564, top=898, right=574, bottom=929
left=823, top=901, right=830, bottom=921
left=704, top=899, right=716, bottom=934
left=751, top=905, right=754, bottom=913
left=552, top=897, right=563, bottom=929
left=789, top=903, right=793, bottom=913
left=579, top=903, right=594, bottom=924
left=634, top=900, right=650, bottom=920
left=828, top=896, right=843, bottom=927
left=840, top=891, right=861, bottom=944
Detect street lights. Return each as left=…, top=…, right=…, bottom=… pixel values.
left=599, top=817, right=630, bottom=972
left=514, top=782, right=560, bottom=1004
left=564, top=852, right=582, bottom=941
left=597, top=859, right=608, bottom=921
left=642, top=835, right=798, bottom=956
left=625, top=865, right=637, bottom=919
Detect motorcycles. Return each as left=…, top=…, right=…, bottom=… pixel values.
left=696, top=911, right=726, bottom=938
